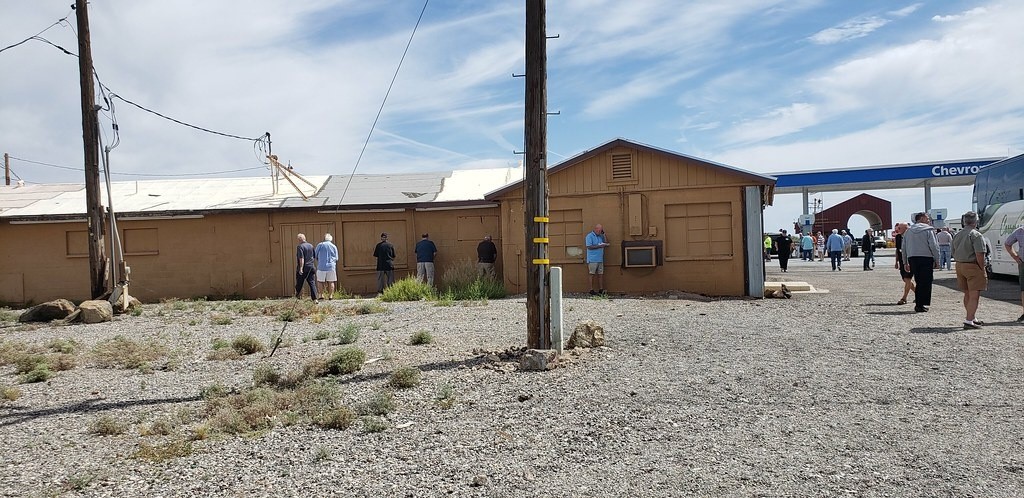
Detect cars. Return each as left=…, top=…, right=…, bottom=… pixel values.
left=874, top=236, right=887, bottom=249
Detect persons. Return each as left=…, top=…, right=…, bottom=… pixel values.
left=894, top=223, right=915, bottom=305
left=315, top=234, right=339, bottom=300
left=901, top=212, right=941, bottom=312
left=826, top=229, right=845, bottom=271
left=951, top=211, right=992, bottom=329
left=763, top=234, right=772, bottom=261
left=476, top=234, right=498, bottom=281
left=861, top=228, right=876, bottom=271
left=841, top=230, right=853, bottom=261
left=373, top=232, right=396, bottom=294
left=414, top=232, right=437, bottom=287
left=1004, top=226, right=1024, bottom=323
left=295, top=234, right=319, bottom=305
left=934, top=227, right=963, bottom=271
left=775, top=229, right=794, bottom=272
left=816, top=231, right=825, bottom=261
left=799, top=232, right=817, bottom=262
left=585, top=223, right=610, bottom=295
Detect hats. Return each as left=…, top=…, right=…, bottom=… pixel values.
left=380, top=232, right=388, bottom=238
left=782, top=230, right=787, bottom=233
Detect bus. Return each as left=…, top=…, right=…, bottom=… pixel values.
left=972, top=154, right=1024, bottom=277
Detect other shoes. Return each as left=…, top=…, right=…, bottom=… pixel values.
left=317, top=298, right=325, bottom=300
left=599, top=288, right=607, bottom=295
left=588, top=289, right=598, bottom=296
left=913, top=305, right=930, bottom=314
left=329, top=297, right=333, bottom=300
left=872, top=261, right=876, bottom=268
left=897, top=298, right=908, bottom=307
left=312, top=298, right=319, bottom=305
left=864, top=268, right=874, bottom=272
left=837, top=266, right=842, bottom=272
left=963, top=319, right=985, bottom=331
left=1016, top=314, right=1024, bottom=326
